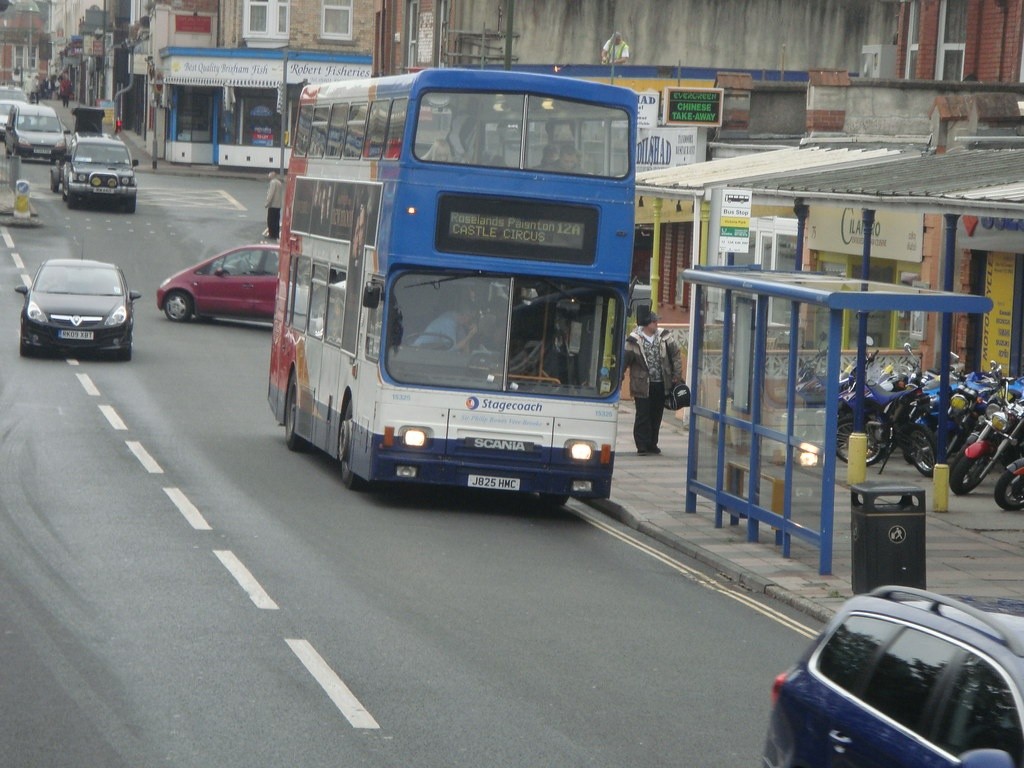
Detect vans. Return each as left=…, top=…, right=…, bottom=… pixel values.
left=6, top=104, right=72, bottom=162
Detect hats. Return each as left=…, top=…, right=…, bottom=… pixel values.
left=650, top=311, right=662, bottom=322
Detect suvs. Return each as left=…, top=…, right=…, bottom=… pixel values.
left=760, top=584, right=1024, bottom=768
left=59, top=131, right=139, bottom=214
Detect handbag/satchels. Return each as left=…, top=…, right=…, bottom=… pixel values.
left=664, top=381, right=690, bottom=410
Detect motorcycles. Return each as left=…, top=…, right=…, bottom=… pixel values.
left=799, top=339, right=1024, bottom=511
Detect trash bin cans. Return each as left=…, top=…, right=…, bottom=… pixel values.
left=850, top=481, right=926, bottom=596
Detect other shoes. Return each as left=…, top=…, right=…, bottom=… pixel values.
left=637, top=448, right=660, bottom=455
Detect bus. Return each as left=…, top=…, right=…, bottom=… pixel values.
left=265, top=66, right=655, bottom=512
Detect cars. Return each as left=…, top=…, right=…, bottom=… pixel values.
left=0, top=84, right=29, bottom=138
left=156, top=243, right=286, bottom=323
left=15, top=256, right=141, bottom=361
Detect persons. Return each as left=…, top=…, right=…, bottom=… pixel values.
left=60, top=74, right=72, bottom=107
left=23, top=75, right=60, bottom=99
left=412, top=307, right=479, bottom=349
left=265, top=171, right=282, bottom=238
left=553, top=146, right=578, bottom=173
left=622, top=310, right=683, bottom=456
left=601, top=32, right=629, bottom=64
left=421, top=139, right=453, bottom=162
